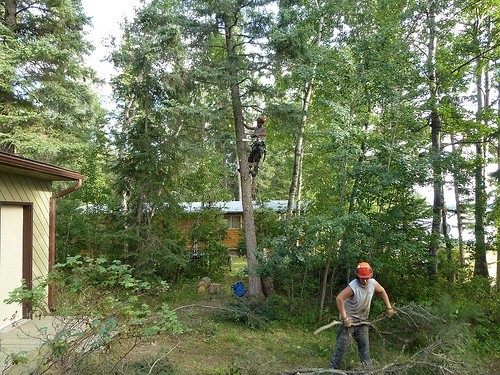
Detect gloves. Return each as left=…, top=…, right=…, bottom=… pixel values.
left=341, top=315, right=353, bottom=328
left=386, top=307, right=394, bottom=318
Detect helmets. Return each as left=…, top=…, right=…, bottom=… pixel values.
left=256, top=116, right=266, bottom=123
left=356, top=261, right=373, bottom=279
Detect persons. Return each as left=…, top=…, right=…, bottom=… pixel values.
left=237, top=116, right=267, bottom=178
left=330, top=261, right=394, bottom=371
